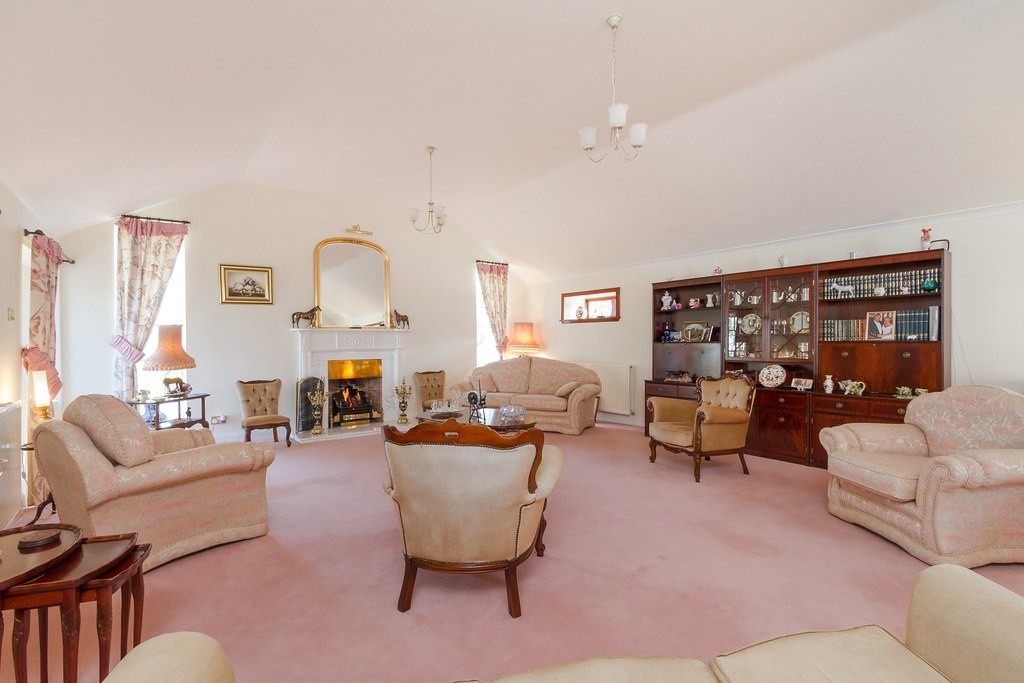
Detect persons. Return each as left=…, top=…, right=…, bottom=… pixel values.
left=869, top=313, right=883, bottom=338
left=877, top=316, right=893, bottom=339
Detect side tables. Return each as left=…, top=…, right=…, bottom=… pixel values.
left=20, top=441, right=60, bottom=526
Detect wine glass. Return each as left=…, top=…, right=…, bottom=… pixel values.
left=771, top=317, right=789, bottom=334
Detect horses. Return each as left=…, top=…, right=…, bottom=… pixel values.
left=292, top=306, right=322, bottom=328
left=394, top=310, right=410, bottom=329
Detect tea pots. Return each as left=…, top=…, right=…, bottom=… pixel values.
left=748, top=294, right=762, bottom=304
left=729, top=288, right=744, bottom=305
left=773, top=288, right=785, bottom=304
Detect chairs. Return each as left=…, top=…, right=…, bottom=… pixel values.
left=415, top=368, right=451, bottom=412
left=236, top=377, right=294, bottom=448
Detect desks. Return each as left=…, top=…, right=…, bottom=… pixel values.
left=0, top=542, right=155, bottom=683
left=128, top=391, right=212, bottom=433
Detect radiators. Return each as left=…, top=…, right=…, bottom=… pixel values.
left=572, top=362, right=637, bottom=418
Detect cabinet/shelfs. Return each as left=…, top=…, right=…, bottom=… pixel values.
left=0, top=401, right=24, bottom=533
left=288, top=327, right=413, bottom=440
left=641, top=246, right=953, bottom=469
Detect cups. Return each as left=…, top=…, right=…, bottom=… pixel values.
left=431, top=398, right=458, bottom=412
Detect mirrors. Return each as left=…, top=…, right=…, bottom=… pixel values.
left=558, top=285, right=622, bottom=325
left=311, top=237, right=396, bottom=332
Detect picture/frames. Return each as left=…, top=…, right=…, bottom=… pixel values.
left=216, top=263, right=274, bottom=307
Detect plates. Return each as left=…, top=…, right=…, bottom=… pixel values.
left=791, top=378, right=812, bottom=389
left=153, top=396, right=166, bottom=402
left=740, top=314, right=762, bottom=334
left=759, top=364, right=786, bottom=387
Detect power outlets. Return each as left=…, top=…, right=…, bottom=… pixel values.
left=210, top=416, right=228, bottom=425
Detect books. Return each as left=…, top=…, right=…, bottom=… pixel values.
left=896, top=305, right=939, bottom=342
left=825, top=266, right=940, bottom=299
left=823, top=318, right=865, bottom=341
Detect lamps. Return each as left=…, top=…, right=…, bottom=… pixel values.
left=506, top=322, right=541, bottom=358
left=32, top=367, right=53, bottom=427
left=407, top=145, right=449, bottom=235
left=578, top=13, right=650, bottom=164
left=142, top=324, right=196, bottom=398
left=306, top=379, right=330, bottom=435
left=395, top=375, right=414, bottom=424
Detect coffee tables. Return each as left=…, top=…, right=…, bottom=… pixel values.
left=413, top=407, right=540, bottom=437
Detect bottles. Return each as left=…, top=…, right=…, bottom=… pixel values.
left=823, top=374, right=834, bottom=394
left=662, top=320, right=670, bottom=342
left=790, top=310, right=810, bottom=333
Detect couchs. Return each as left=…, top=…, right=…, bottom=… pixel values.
left=380, top=417, right=564, bottom=620
left=91, top=558, right=1024, bottom=683
left=451, top=357, right=602, bottom=436
left=819, top=383, right=1024, bottom=571
left=32, top=394, right=276, bottom=577
left=645, top=370, right=757, bottom=483
